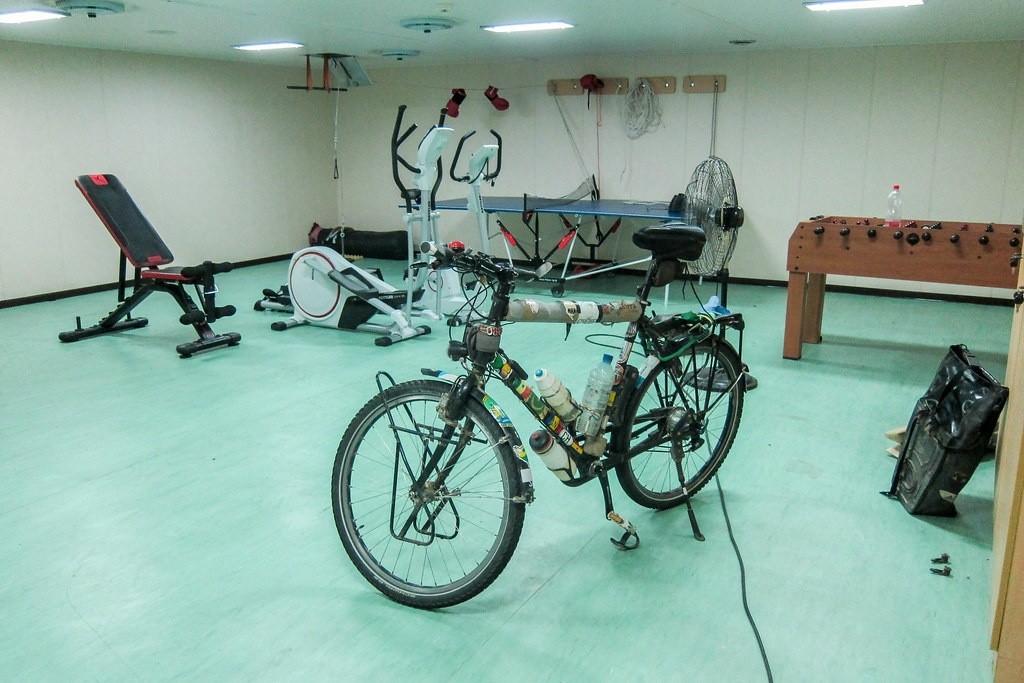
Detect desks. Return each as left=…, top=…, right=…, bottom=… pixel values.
left=399, top=195, right=703, bottom=300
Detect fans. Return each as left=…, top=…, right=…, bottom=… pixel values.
left=681, top=153, right=757, bottom=391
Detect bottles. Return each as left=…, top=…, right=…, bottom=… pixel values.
left=605, top=365, right=640, bottom=427
left=578, top=353, right=617, bottom=436
left=529, top=430, right=577, bottom=481
left=887, top=185, right=902, bottom=228
left=533, top=368, right=580, bottom=421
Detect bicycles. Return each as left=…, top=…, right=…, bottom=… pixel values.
left=331, top=221, right=751, bottom=610
